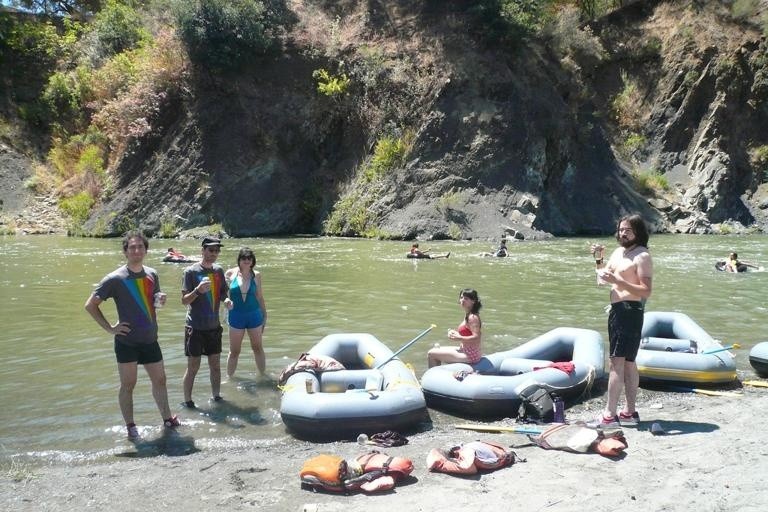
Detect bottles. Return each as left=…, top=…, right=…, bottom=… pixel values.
left=594, top=244, right=605, bottom=269
left=551, top=396, right=566, bottom=426
left=202, top=277, right=210, bottom=292
left=305, top=377, right=316, bottom=393
left=154, top=290, right=167, bottom=310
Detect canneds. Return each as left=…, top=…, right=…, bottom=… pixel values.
left=154, top=293, right=166, bottom=308
left=594, top=244, right=602, bottom=258
left=306, top=378, right=313, bottom=393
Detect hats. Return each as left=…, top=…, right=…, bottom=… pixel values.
left=201, top=236, right=224, bottom=248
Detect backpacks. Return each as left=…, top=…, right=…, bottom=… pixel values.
left=518, top=384, right=556, bottom=423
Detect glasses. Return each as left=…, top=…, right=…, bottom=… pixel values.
left=241, top=255, right=253, bottom=261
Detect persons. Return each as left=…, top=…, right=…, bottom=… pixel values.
left=83, top=232, right=182, bottom=443
left=181, top=235, right=233, bottom=411
left=223, top=246, right=271, bottom=380
left=478, top=239, right=508, bottom=258
left=411, top=243, right=450, bottom=259
left=426, top=288, right=482, bottom=370
left=590, top=213, right=652, bottom=428
left=724, top=252, right=738, bottom=273
left=167, top=246, right=184, bottom=258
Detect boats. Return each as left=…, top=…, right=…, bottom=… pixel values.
left=279, top=332, right=428, bottom=437
left=714, top=261, right=745, bottom=273
left=748, top=341, right=767, bottom=378
left=405, top=253, right=429, bottom=259
left=635, top=307, right=739, bottom=387
left=164, top=257, right=185, bottom=264
left=421, top=325, right=608, bottom=417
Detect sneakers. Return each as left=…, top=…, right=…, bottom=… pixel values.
left=126, top=423, right=140, bottom=440
left=212, top=396, right=223, bottom=402
left=617, top=411, right=641, bottom=426
left=586, top=413, right=621, bottom=430
left=164, top=414, right=182, bottom=427
left=184, top=400, right=195, bottom=409
